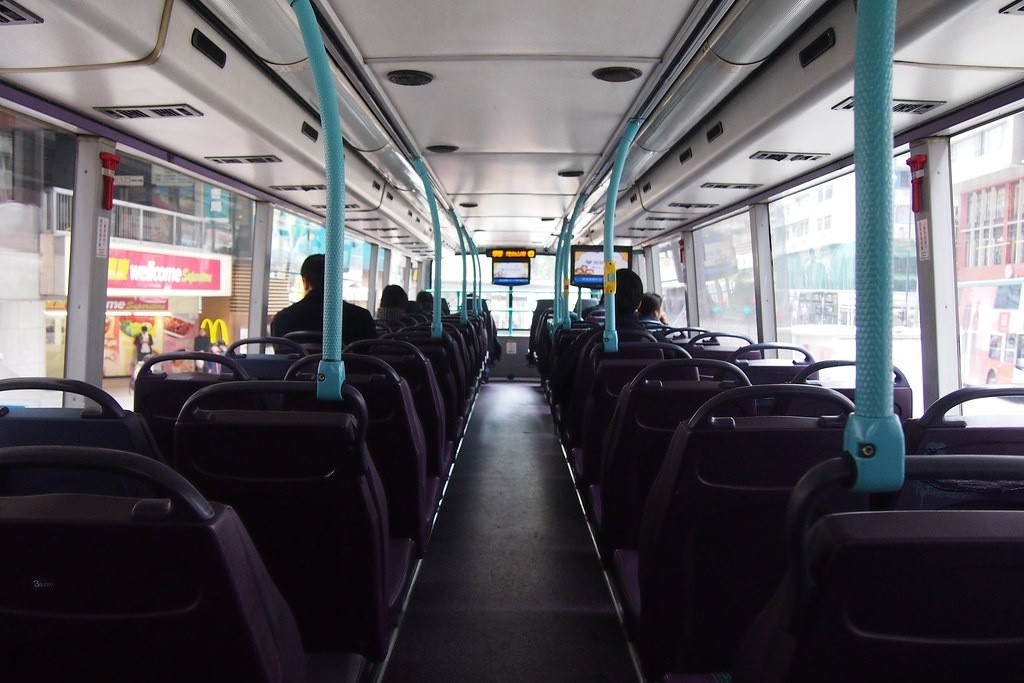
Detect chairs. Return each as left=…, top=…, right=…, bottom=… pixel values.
left=534, top=308, right=1024, bottom=683
left=0, top=310, right=489, bottom=683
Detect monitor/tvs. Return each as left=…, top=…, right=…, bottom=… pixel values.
left=492, top=257, right=531, bottom=286
left=570, top=245, right=633, bottom=290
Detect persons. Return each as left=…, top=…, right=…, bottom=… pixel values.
left=416, top=292, right=434, bottom=313
left=372, top=284, right=409, bottom=323
left=556, top=268, right=669, bottom=406
left=582, top=291, right=605, bottom=320
left=637, top=292, right=669, bottom=328
left=270, top=252, right=380, bottom=355
left=130, top=325, right=155, bottom=387
left=194, top=328, right=230, bottom=375
